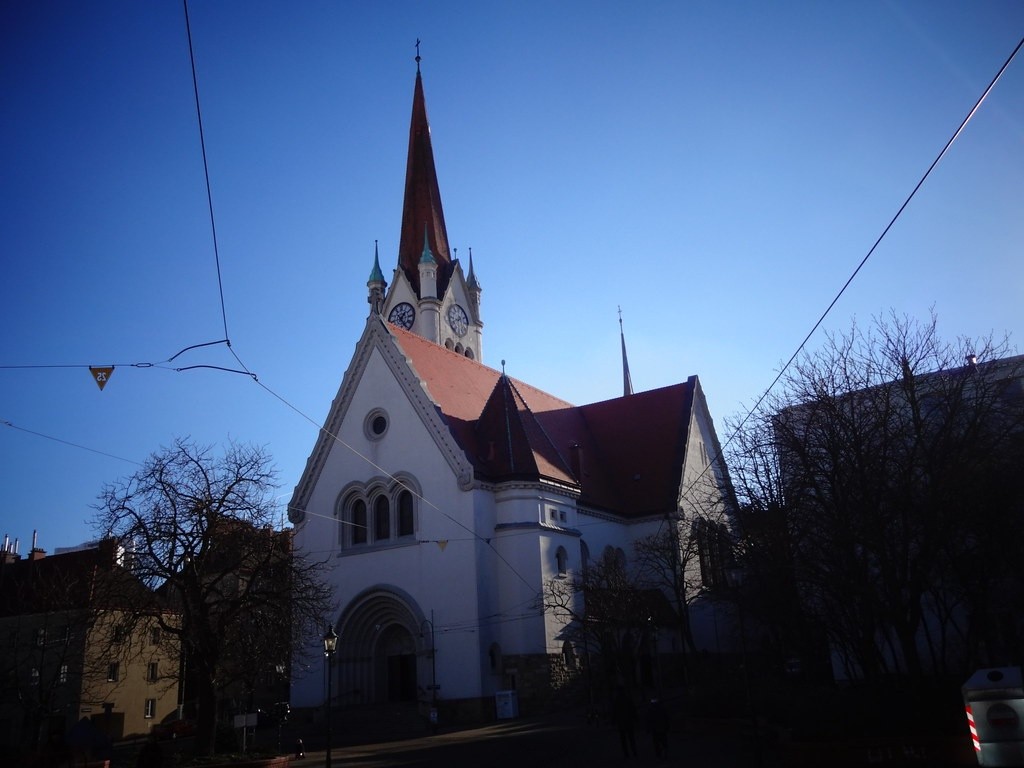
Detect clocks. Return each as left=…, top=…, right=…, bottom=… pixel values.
left=447, top=304, right=469, bottom=338
left=388, top=302, right=415, bottom=331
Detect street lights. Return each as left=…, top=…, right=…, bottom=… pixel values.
left=321, top=622, right=340, bottom=768
left=419, top=609, right=442, bottom=728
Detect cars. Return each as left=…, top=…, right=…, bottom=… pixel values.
left=153, top=718, right=200, bottom=740
